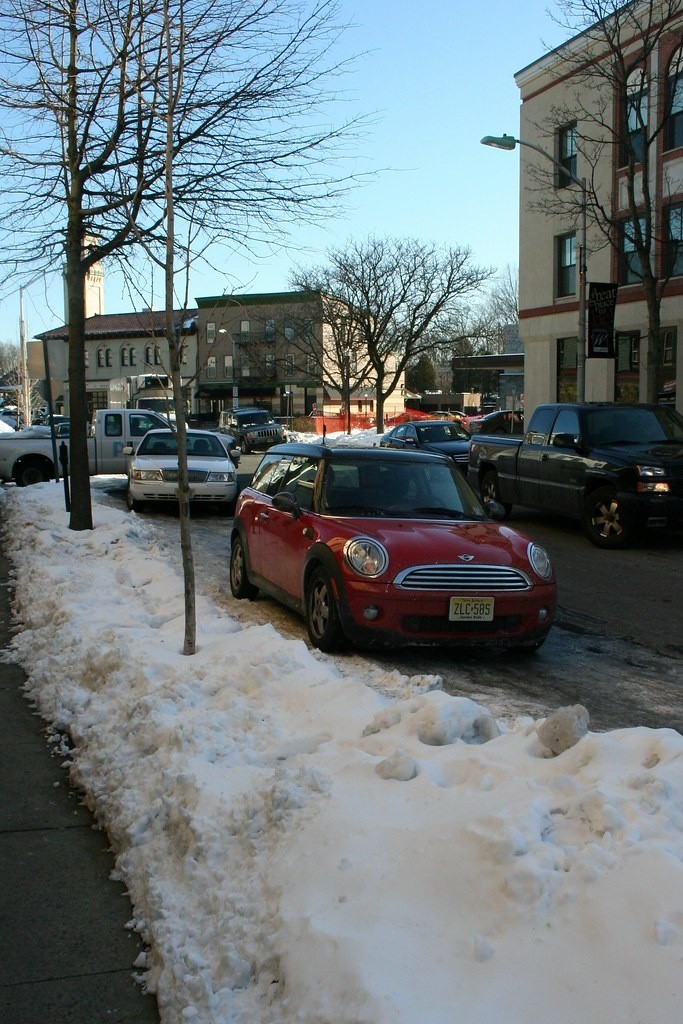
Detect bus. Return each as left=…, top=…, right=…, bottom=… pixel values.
left=0, top=385, right=23, bottom=407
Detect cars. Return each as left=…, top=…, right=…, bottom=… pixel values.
left=470, top=409, right=525, bottom=435
left=228, top=439, right=558, bottom=657
left=429, top=410, right=453, bottom=417
left=378, top=419, right=471, bottom=466
left=122, top=427, right=239, bottom=511
left=2, top=404, right=70, bottom=439
left=450, top=411, right=467, bottom=417
left=383, top=412, right=422, bottom=427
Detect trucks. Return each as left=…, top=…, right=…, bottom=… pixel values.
left=123, top=374, right=191, bottom=431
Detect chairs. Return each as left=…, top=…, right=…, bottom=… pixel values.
left=368, top=471, right=414, bottom=512
left=152, top=439, right=210, bottom=455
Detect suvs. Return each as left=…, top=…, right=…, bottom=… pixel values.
left=218, top=406, right=286, bottom=454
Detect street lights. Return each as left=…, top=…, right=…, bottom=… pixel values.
left=218, top=327, right=239, bottom=410
left=485, top=129, right=587, bottom=402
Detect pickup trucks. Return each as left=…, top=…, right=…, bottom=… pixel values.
left=0, top=409, right=176, bottom=488
left=466, top=403, right=683, bottom=549
